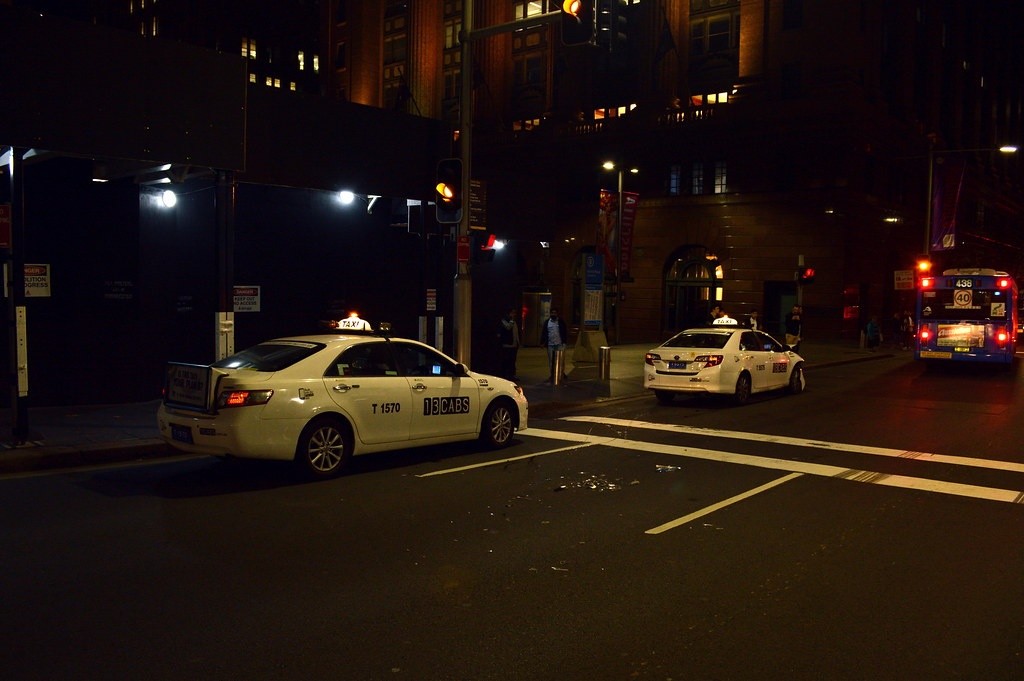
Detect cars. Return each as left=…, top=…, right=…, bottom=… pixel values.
left=156, top=314, right=531, bottom=476
left=643, top=325, right=808, bottom=402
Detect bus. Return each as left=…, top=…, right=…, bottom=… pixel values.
left=915, top=268, right=1018, bottom=379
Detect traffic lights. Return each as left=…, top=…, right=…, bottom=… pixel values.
left=917, top=255, right=932, bottom=291
left=471, top=231, right=497, bottom=252
left=434, top=157, right=463, bottom=224
left=800, top=267, right=816, bottom=285
left=598, top=0, right=629, bottom=54
left=560, top=0, right=598, bottom=46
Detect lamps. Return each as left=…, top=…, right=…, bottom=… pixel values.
left=330, top=189, right=368, bottom=210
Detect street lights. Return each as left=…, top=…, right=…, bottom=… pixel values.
left=1, top=146, right=43, bottom=440
left=604, top=160, right=640, bottom=346
left=925, top=143, right=1019, bottom=259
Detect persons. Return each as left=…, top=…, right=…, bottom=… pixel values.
left=744, top=308, right=764, bottom=331
left=540, top=308, right=568, bottom=383
left=784, top=306, right=804, bottom=353
left=500, top=308, right=521, bottom=381
left=706, top=305, right=720, bottom=326
